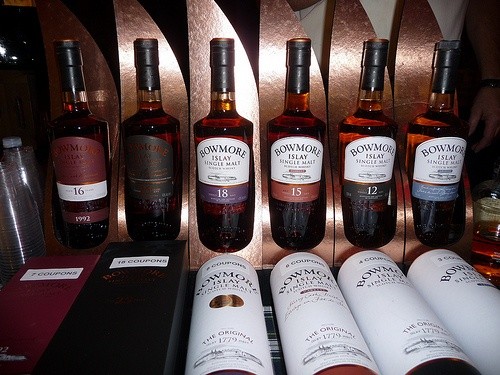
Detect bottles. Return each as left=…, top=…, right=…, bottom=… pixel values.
left=270, top=251, right=378, bottom=375
left=120, top=38, right=181, bottom=245
left=44, top=37, right=110, bottom=250
left=337, top=37, right=400, bottom=250
left=337, top=250, right=482, bottom=375
left=405, top=39, right=468, bottom=247
left=193, top=37, right=256, bottom=253
left=407, top=250, right=500, bottom=374
left=184, top=254, right=276, bottom=375
left=1, top=136, right=24, bottom=164
left=266, top=37, right=327, bottom=252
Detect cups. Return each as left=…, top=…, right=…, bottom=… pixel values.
left=0, top=147, right=46, bottom=285
left=468, top=156, right=500, bottom=244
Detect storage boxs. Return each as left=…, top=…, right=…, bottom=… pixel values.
left=0, top=238, right=189, bottom=375
